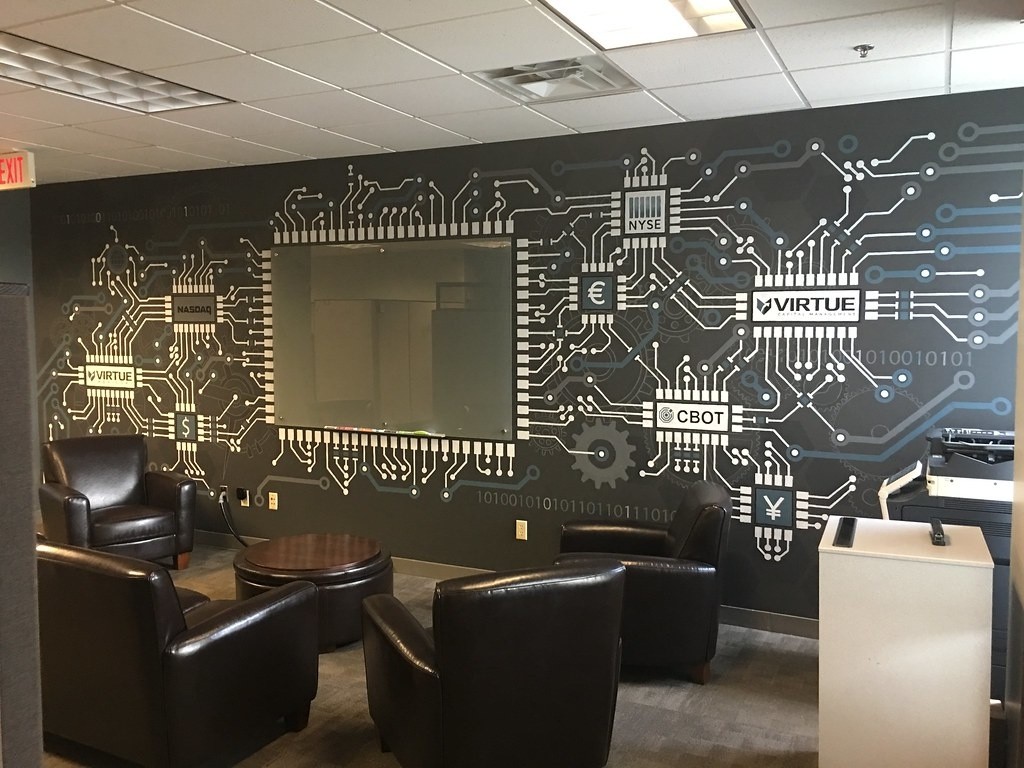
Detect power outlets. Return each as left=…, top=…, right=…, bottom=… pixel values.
left=269, top=492, right=278, bottom=510
left=240, top=490, right=249, bottom=507
left=220, top=485, right=229, bottom=504
left=515, top=519, right=529, bottom=542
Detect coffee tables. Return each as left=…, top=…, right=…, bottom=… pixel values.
left=233, top=534, right=392, bottom=655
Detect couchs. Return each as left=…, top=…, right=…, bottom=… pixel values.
left=539, top=480, right=732, bottom=685
left=38, top=434, right=197, bottom=570
left=36, top=540, right=318, bottom=768
left=361, top=556, right=626, bottom=768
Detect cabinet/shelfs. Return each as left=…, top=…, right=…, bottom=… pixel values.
left=816, top=515, right=996, bottom=767
